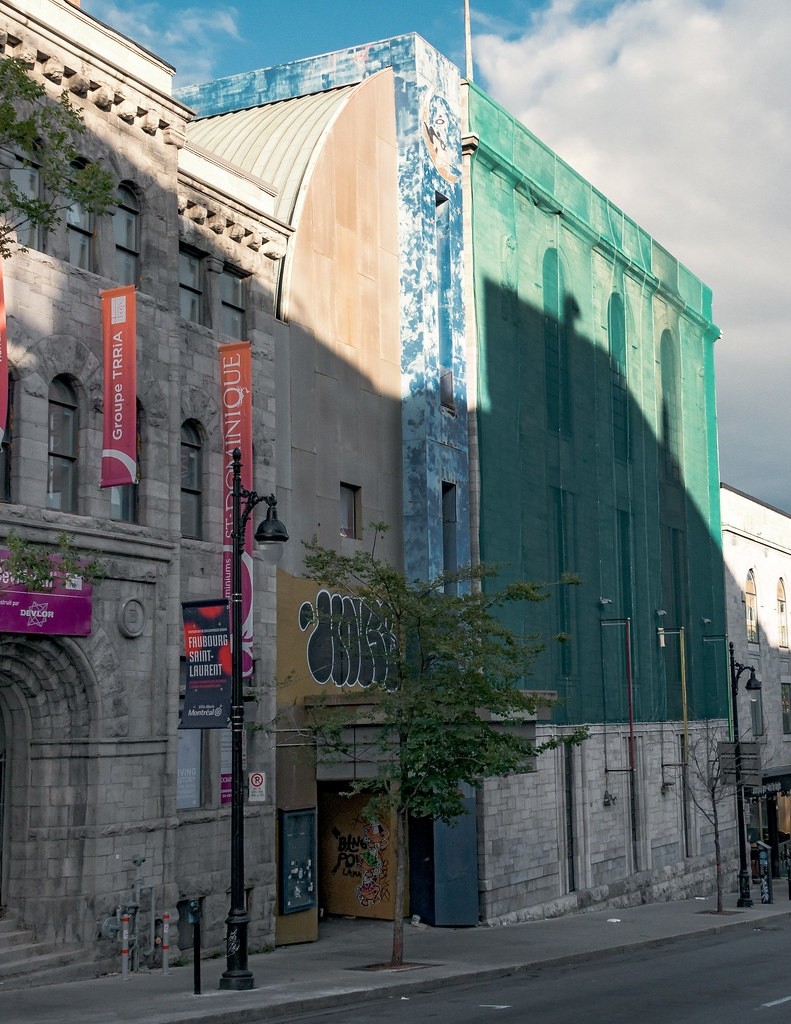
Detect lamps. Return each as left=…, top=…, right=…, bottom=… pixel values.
left=655, top=608, right=667, bottom=616
left=599, top=595, right=613, bottom=605
left=604, top=790, right=618, bottom=806
left=661, top=781, right=675, bottom=795
left=701, top=616, right=711, bottom=624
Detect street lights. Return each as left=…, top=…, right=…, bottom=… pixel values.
left=219, top=447, right=289, bottom=992
left=727, top=641, right=762, bottom=908
